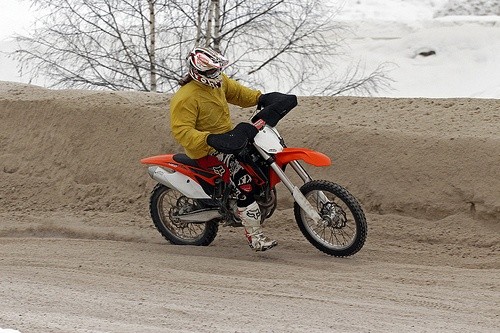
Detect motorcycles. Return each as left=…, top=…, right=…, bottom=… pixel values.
left=139, top=95, right=368, bottom=257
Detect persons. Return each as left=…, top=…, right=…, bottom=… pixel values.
left=170, top=48, right=297, bottom=251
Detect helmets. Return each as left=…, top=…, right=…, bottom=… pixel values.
left=186, top=47, right=229, bottom=88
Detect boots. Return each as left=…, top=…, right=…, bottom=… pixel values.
left=237, top=201, right=278, bottom=251
